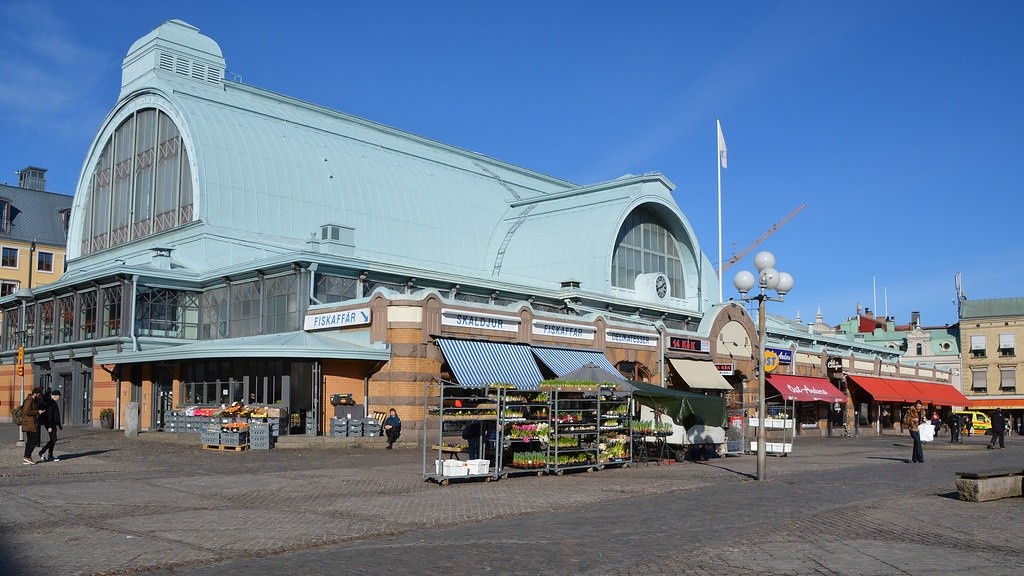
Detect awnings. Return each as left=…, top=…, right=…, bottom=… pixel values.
left=763, top=373, right=848, bottom=402
left=433, top=337, right=632, bottom=393
left=849, top=374, right=973, bottom=406
left=93, top=332, right=391, bottom=374
left=667, top=352, right=735, bottom=391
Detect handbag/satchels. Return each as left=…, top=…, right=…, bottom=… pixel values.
left=918, top=421, right=935, bottom=442
left=11, top=399, right=24, bottom=426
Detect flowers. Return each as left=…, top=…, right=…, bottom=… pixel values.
left=510, top=422, right=555, bottom=444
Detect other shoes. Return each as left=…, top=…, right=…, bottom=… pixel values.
left=22, top=457, right=35, bottom=465
left=911, top=458, right=925, bottom=463
left=987, top=445, right=994, bottom=449
left=1000, top=447, right=1006, bottom=450
left=47, top=455, right=60, bottom=462
left=385, top=446, right=392, bottom=450
left=36, top=452, right=46, bottom=461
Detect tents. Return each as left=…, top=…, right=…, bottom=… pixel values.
left=627, top=378, right=728, bottom=461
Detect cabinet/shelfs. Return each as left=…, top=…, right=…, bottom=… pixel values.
left=596, top=385, right=634, bottom=471
left=745, top=393, right=795, bottom=458
left=423, top=380, right=501, bottom=486
left=723, top=392, right=744, bottom=458
left=483, top=383, right=554, bottom=480
left=550, top=387, right=600, bottom=477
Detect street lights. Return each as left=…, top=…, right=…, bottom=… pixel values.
left=13, top=288, right=35, bottom=447
left=732, top=251, right=794, bottom=478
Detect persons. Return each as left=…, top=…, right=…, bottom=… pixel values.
left=902, top=400, right=927, bottom=463
left=931, top=407, right=1021, bottom=450
left=35, top=390, right=63, bottom=462
left=382, top=408, right=402, bottom=450
left=20, top=387, right=42, bottom=465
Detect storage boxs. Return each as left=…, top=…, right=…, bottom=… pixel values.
left=786, top=420, right=793, bottom=428
left=750, top=442, right=757, bottom=451
left=802, top=421, right=817, bottom=429
left=749, top=417, right=760, bottom=426
left=690, top=448, right=713, bottom=459
left=330, top=393, right=353, bottom=406
left=765, top=442, right=771, bottom=451
left=784, top=443, right=792, bottom=452
left=305, top=424, right=317, bottom=430
left=771, top=443, right=784, bottom=452
left=291, top=413, right=300, bottom=427
left=163, top=402, right=288, bottom=450
left=306, top=417, right=315, bottom=424
left=818, top=418, right=828, bottom=429
left=772, top=420, right=785, bottom=428
left=306, top=430, right=317, bottom=436
left=329, top=418, right=381, bottom=437
left=465, top=458, right=491, bottom=475
left=434, top=459, right=467, bottom=476
left=305, top=411, right=312, bottom=418
left=764, top=419, right=772, bottom=427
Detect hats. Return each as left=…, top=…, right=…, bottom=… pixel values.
left=51, top=390, right=63, bottom=396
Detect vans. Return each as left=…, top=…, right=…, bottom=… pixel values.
left=954, top=411, right=993, bottom=436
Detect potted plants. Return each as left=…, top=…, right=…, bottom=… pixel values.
left=557, top=411, right=588, bottom=424
left=558, top=434, right=581, bottom=450
left=506, top=395, right=523, bottom=404
left=600, top=419, right=618, bottom=429
left=631, top=420, right=672, bottom=436
left=551, top=439, right=555, bottom=450
left=600, top=381, right=624, bottom=391
left=558, top=425, right=595, bottom=433
left=623, top=418, right=629, bottom=428
left=476, top=400, right=497, bottom=408
left=599, top=431, right=631, bottom=462
left=727, top=427, right=743, bottom=451
left=428, top=409, right=440, bottom=419
left=601, top=404, right=628, bottom=418
left=526, top=393, right=531, bottom=403
left=550, top=456, right=555, bottom=468
left=505, top=409, right=524, bottom=419
left=531, top=394, right=549, bottom=405
left=99, top=408, right=114, bottom=430
left=489, top=383, right=517, bottom=390
left=506, top=451, right=547, bottom=470
left=530, top=407, right=549, bottom=420
left=443, top=410, right=497, bottom=420
left=540, top=378, right=599, bottom=391
left=557, top=452, right=596, bottom=468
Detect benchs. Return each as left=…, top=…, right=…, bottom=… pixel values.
left=955, top=466, right=1024, bottom=502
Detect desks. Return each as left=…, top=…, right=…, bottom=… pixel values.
left=431, top=445, right=469, bottom=460
left=682, top=443, right=724, bottom=462
left=633, top=433, right=672, bottom=466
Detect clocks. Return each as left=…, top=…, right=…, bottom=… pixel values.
left=656, top=276, right=667, bottom=300
